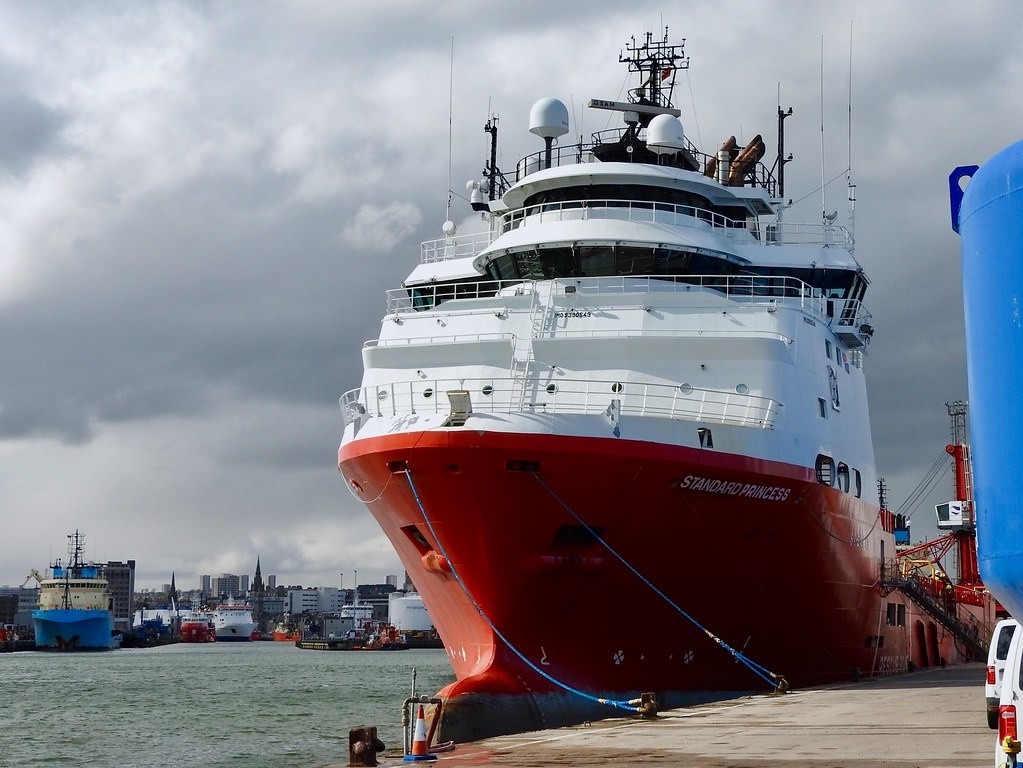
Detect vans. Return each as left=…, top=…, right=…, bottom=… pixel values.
left=985, top=619, right=1022, bottom=729
left=995, top=625, right=1022, bottom=768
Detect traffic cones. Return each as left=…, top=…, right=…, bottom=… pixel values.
left=402, top=704, right=438, bottom=761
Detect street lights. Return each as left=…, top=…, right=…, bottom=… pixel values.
left=340, top=573, right=342, bottom=613
left=354, top=570, right=356, bottom=625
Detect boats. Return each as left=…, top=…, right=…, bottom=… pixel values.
left=272, top=611, right=301, bottom=641
left=31, top=528, right=122, bottom=648
left=295, top=611, right=409, bottom=651
left=124, top=597, right=192, bottom=648
left=333, top=23, right=1009, bottom=749
left=180, top=592, right=215, bottom=641
left=215, top=591, right=258, bottom=641
